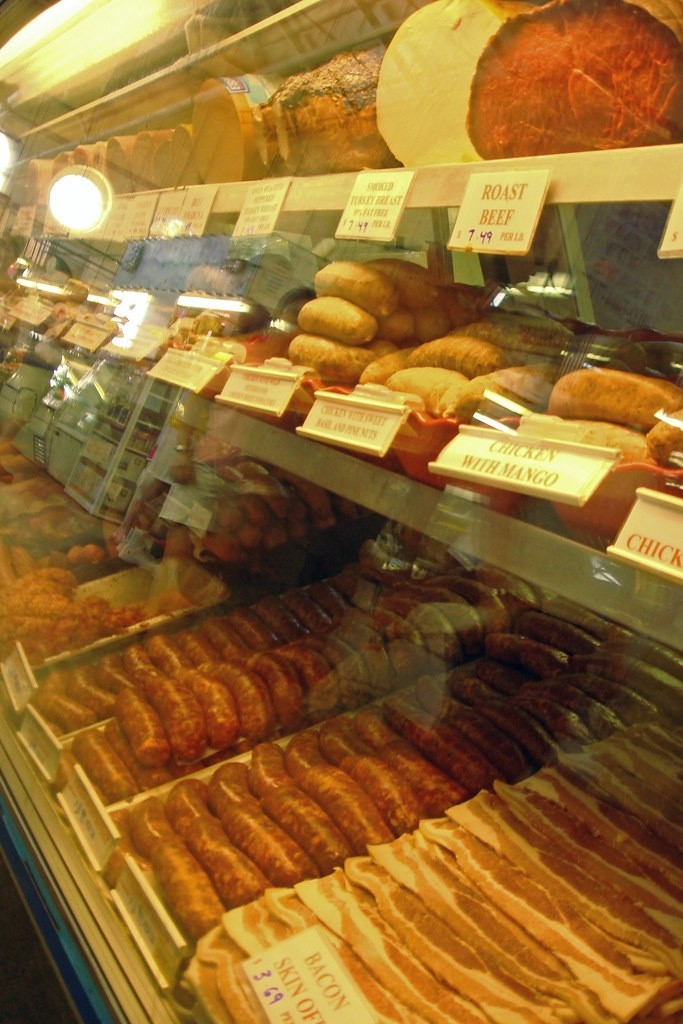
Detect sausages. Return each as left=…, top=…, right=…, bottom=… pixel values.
left=288, top=257, right=683, bottom=468
left=30, top=452, right=683, bottom=933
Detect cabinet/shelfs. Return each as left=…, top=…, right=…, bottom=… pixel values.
left=0, top=0, right=683, bottom=1024
